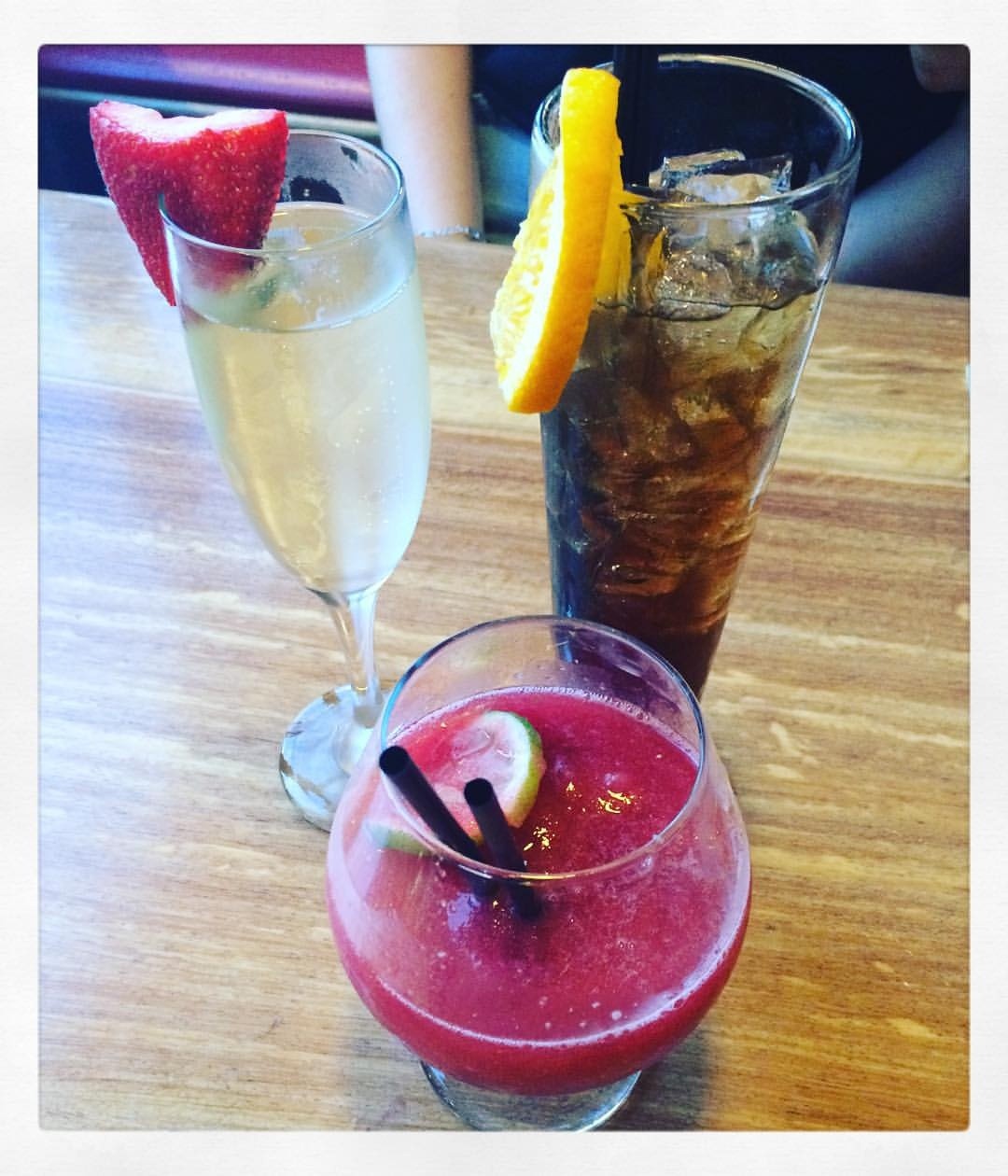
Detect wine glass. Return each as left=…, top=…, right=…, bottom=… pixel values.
left=157, top=128, right=432, bottom=833
left=326, top=613, right=753, bottom=1131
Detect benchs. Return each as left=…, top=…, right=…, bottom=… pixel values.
left=39, top=45, right=379, bottom=137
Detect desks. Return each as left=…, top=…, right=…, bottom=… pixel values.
left=38, top=188, right=968, bottom=1132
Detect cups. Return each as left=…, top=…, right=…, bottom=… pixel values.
left=530, top=52, right=863, bottom=702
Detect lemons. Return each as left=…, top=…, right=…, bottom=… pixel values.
left=486, top=66, right=635, bottom=415
left=359, top=711, right=545, bottom=856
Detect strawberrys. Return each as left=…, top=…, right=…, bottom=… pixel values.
left=88, top=100, right=290, bottom=307
